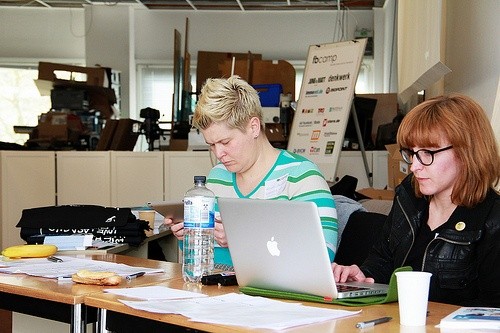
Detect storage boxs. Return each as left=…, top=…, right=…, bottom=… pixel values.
left=386, top=145, right=412, bottom=190
left=39, top=88, right=97, bottom=146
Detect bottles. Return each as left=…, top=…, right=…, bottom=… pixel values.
left=182, top=176, right=216, bottom=282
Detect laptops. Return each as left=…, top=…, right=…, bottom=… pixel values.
left=219, top=198, right=389, bottom=298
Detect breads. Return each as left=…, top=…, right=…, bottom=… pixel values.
left=72, top=269, right=121, bottom=286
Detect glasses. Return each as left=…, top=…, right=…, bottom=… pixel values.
left=399, top=146, right=452, bottom=166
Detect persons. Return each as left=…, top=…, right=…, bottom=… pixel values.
left=164, top=74, right=338, bottom=268
left=329, top=96, right=500, bottom=307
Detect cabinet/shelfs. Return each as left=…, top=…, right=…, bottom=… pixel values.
left=0, top=151, right=388, bottom=255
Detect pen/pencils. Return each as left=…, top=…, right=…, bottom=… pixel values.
left=125, top=272, right=145, bottom=280
left=354, top=317, right=392, bottom=328
left=47, top=255, right=62, bottom=262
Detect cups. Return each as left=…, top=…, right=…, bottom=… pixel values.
left=394, top=271, right=432, bottom=333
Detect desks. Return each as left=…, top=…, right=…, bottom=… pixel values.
left=0, top=205, right=500, bottom=333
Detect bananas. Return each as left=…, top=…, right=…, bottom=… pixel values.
left=1, top=244, right=57, bottom=257
